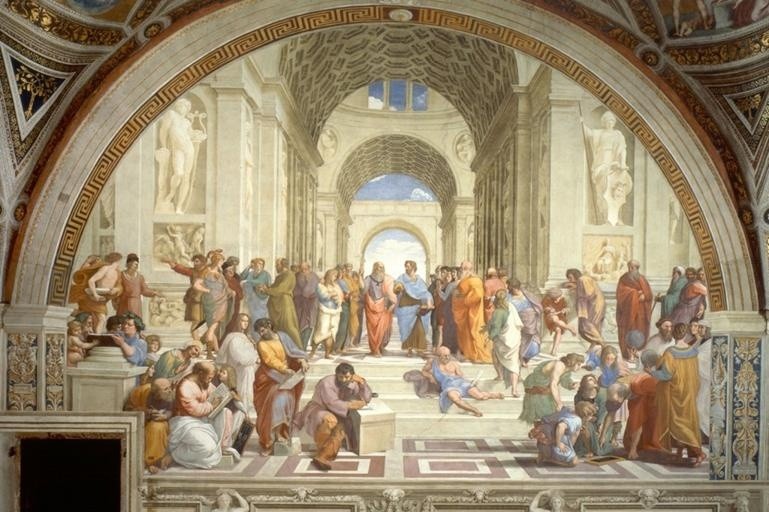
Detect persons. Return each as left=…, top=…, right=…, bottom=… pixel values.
left=581, top=111, right=633, bottom=224
left=67, top=248, right=711, bottom=469
left=159, top=97, right=198, bottom=214
